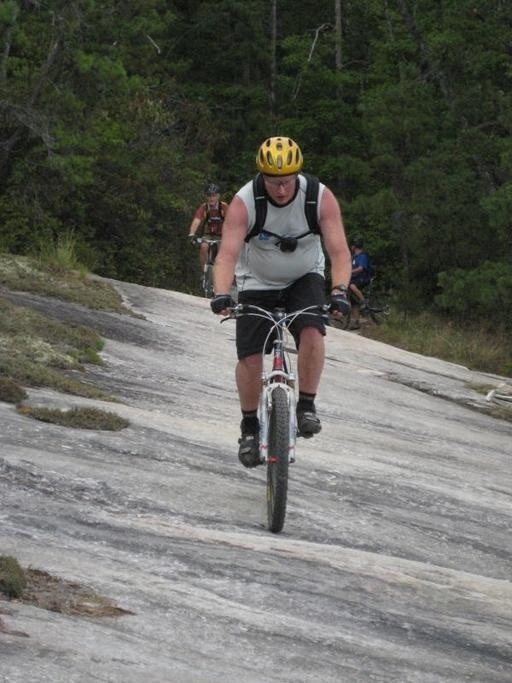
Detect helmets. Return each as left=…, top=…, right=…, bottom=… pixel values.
left=256, top=136, right=304, bottom=176
left=204, top=183, right=220, bottom=195
left=350, top=234, right=364, bottom=249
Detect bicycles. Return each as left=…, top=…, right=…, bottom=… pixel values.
left=330, top=273, right=399, bottom=329
left=192, top=235, right=222, bottom=299
left=219, top=302, right=342, bottom=533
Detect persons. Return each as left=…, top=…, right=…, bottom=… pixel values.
left=209, top=132, right=354, bottom=466
left=348, top=234, right=374, bottom=311
left=186, top=182, right=229, bottom=283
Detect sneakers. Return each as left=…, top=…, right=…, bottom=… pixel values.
left=237, top=419, right=261, bottom=468
left=358, top=302, right=369, bottom=312
left=296, top=402, right=322, bottom=434
